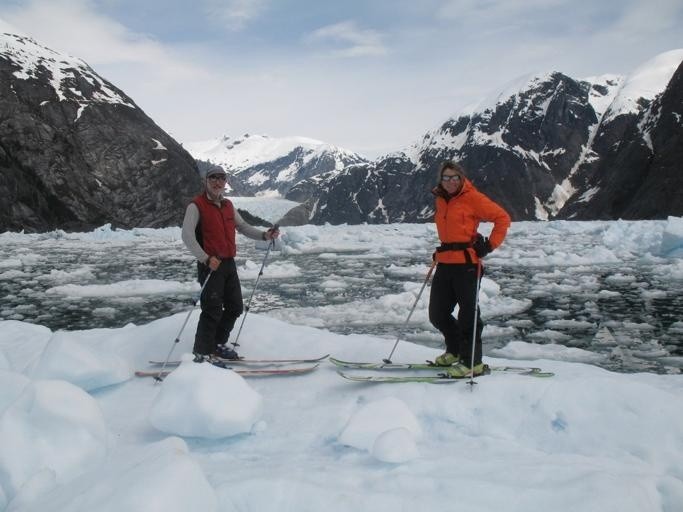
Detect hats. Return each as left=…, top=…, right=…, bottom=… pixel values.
left=206, top=166, right=226, bottom=178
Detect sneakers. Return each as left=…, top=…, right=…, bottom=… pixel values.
left=193, top=352, right=224, bottom=368
left=215, top=344, right=237, bottom=358
left=436, top=351, right=461, bottom=366
left=448, top=360, right=484, bottom=376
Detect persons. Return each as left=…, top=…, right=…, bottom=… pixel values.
left=425, top=160, right=511, bottom=380
left=178, top=162, right=281, bottom=368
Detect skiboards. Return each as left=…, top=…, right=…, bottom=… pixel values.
left=329, top=358, right=555, bottom=381
left=134, top=354, right=331, bottom=377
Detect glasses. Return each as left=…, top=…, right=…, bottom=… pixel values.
left=209, top=175, right=226, bottom=183
left=442, top=175, right=459, bottom=181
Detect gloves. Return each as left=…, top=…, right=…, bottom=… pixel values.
left=475, top=236, right=491, bottom=257
